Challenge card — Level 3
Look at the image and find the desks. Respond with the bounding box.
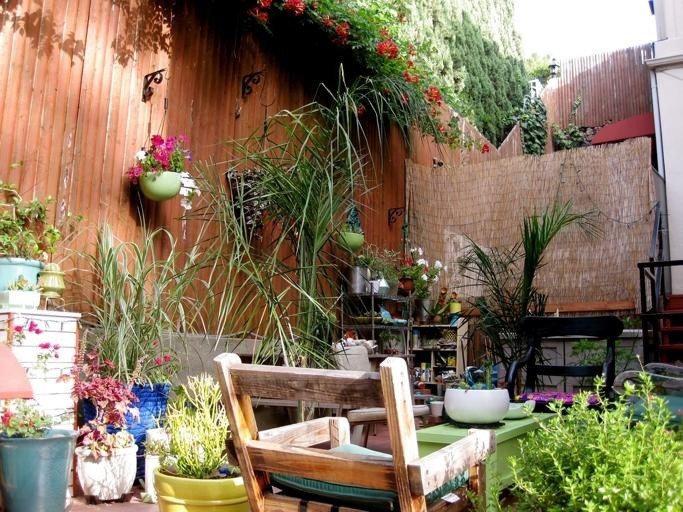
[415,411,561,511]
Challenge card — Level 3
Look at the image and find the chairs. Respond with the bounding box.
[505,314,626,424]
[331,343,432,448]
[212,351,498,512]
[612,361,682,437]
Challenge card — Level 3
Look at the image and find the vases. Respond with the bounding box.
[81,379,171,487]
[0,428,73,512]
[398,276,415,298]
[445,387,510,425]
[75,444,139,502]
[139,170,183,202]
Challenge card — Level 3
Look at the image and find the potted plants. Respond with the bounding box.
[137,370,252,512]
[336,219,366,253]
[2,273,43,310]
[0,157,84,290]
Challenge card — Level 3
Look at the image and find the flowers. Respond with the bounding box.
[47,197,218,391]
[0,409,51,439]
[400,246,449,299]
[124,132,194,186]
[59,341,142,460]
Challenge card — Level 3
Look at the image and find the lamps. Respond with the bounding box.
[0,342,33,415]
